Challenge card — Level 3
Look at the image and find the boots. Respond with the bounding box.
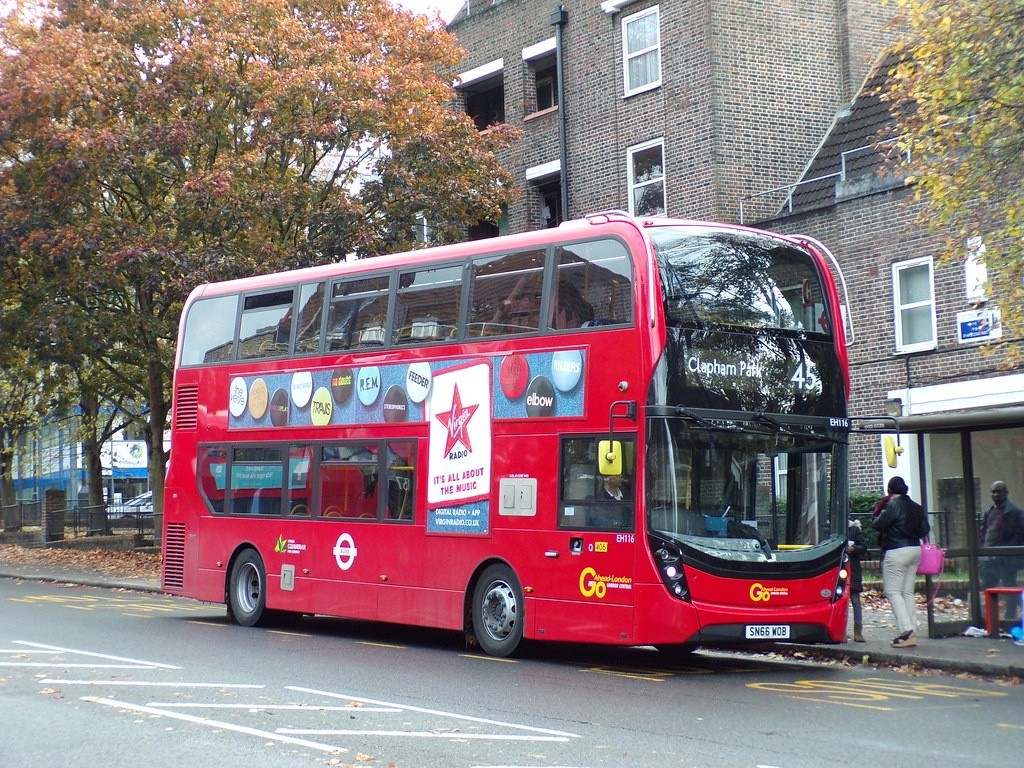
[854,624,866,642]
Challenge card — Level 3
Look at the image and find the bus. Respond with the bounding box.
[159,210,904,659]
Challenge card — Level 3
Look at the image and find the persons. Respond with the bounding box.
[872,476,931,648]
[980,480,1024,619]
[846,519,868,642]
[590,474,634,527]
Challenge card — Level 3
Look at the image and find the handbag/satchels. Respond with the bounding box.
[917,536,943,573]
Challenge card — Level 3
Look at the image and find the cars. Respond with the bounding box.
[108,491,154,521]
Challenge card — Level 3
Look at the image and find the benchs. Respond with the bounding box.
[984,585,1024,637]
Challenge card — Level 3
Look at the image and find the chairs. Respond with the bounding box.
[285,505,377,520]
[222,323,506,360]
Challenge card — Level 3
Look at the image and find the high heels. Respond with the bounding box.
[891,630,917,648]
